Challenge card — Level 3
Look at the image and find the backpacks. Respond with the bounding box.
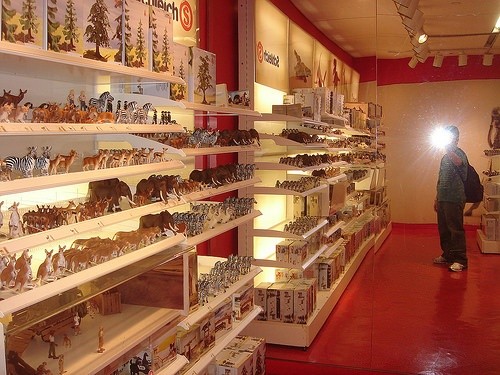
[452,148,483,202]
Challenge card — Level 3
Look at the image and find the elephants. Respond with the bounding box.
[288,132,312,144]
[189,162,237,186]
[139,210,179,233]
[87,174,182,213]
[215,128,263,147]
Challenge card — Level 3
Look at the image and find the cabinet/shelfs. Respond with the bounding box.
[375,104,394,256]
[475,147,500,254]
[247,110,331,354]
[346,120,375,259]
[101,85,267,375]
[0,37,194,375]
[287,115,351,302]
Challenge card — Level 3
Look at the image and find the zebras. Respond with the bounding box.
[3,146,52,178]
[89,92,156,123]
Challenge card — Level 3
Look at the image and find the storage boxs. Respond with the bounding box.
[117,202,268,375]
[256,232,351,325]
[481,214,497,239]
[276,86,367,123]
[0,0,218,115]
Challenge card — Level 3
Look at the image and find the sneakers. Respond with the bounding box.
[434,255,450,263]
[449,262,465,271]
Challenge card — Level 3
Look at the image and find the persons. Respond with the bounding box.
[433,125,468,272]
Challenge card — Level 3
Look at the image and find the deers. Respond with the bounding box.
[0,88,27,109]
[50,148,169,175]
[22,200,86,235]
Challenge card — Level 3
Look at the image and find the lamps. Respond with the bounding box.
[390,0,495,69]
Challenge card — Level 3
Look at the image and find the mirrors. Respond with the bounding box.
[206,0,500,375]
